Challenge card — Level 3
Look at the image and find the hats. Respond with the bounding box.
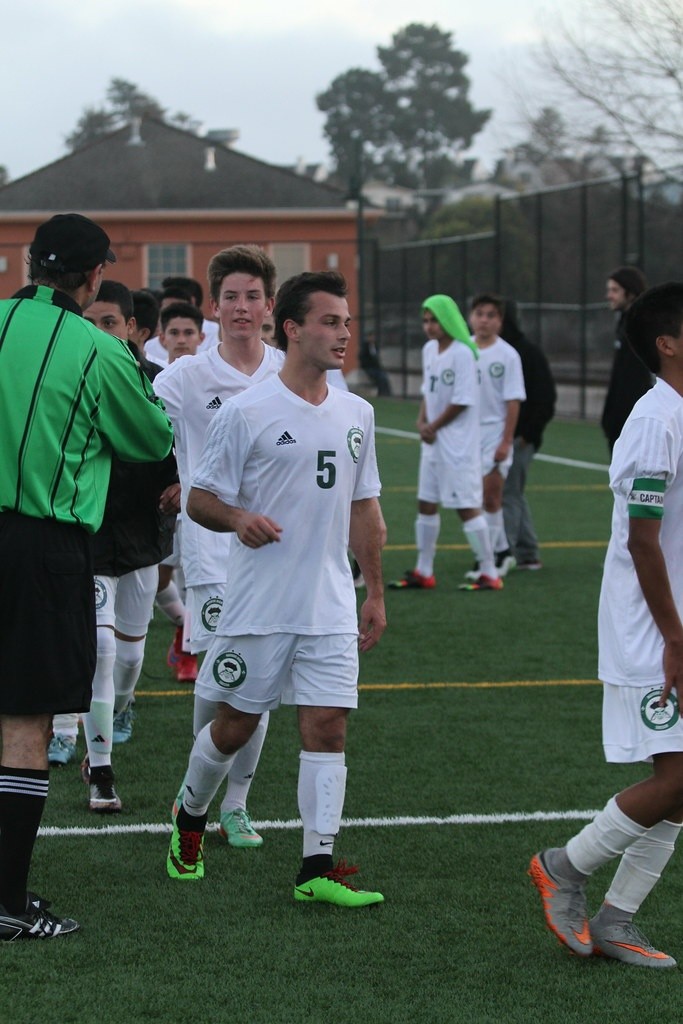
[28,213,118,268]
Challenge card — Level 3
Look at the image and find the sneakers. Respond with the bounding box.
[177,651,199,683]
[113,699,133,745]
[81,752,92,783]
[525,847,593,956]
[218,807,264,848]
[293,848,385,907]
[464,549,516,579]
[0,903,79,941]
[389,569,435,590]
[167,814,204,881]
[48,733,78,764]
[171,782,185,821]
[87,765,122,812]
[588,902,677,969]
[167,626,182,667]
[457,573,504,592]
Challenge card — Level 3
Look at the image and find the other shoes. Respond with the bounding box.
[515,558,542,571]
[354,568,368,589]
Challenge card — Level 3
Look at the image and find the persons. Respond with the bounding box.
[0,212,287,945]
[600,270,659,464]
[504,299,559,573]
[526,281,683,970]
[164,269,388,909]
[392,292,504,590]
[468,295,517,579]
[359,331,393,397]
